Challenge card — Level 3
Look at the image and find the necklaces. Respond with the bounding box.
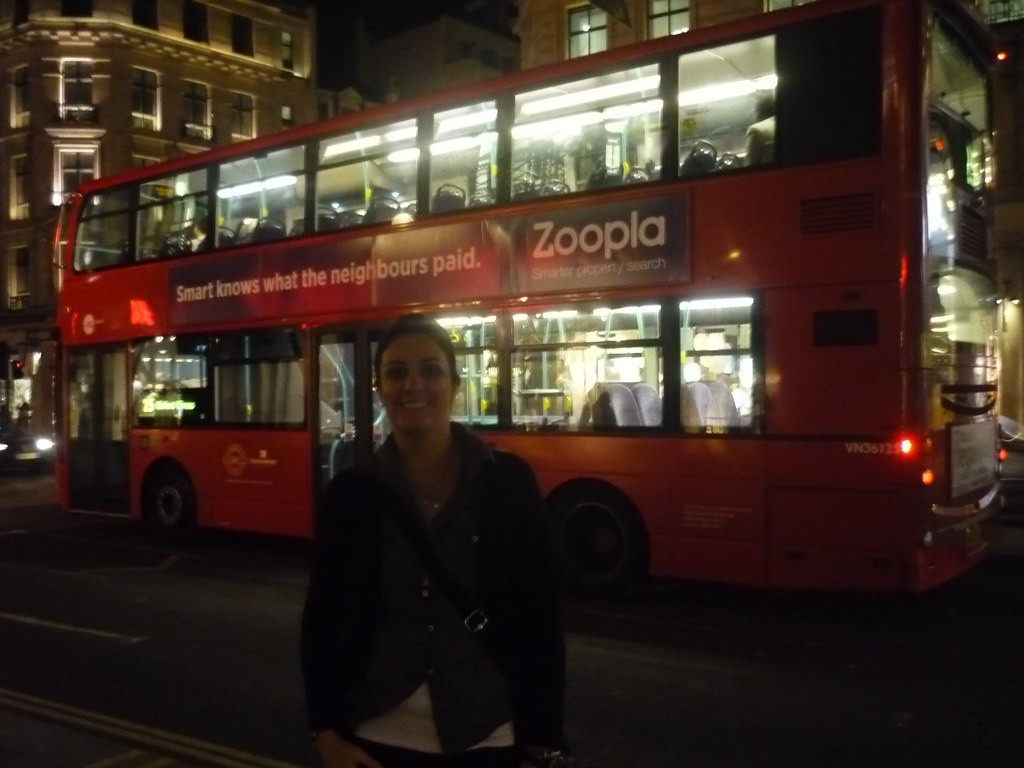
[419,497,442,510]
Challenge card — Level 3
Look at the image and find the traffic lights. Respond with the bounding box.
[10,361,24,380]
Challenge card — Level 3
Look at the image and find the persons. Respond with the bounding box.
[744,90,776,167]
[298,312,573,768]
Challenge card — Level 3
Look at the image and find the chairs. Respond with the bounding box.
[708,382,740,426]
[543,178,571,196]
[600,383,643,427]
[631,383,662,427]
[682,381,719,425]
[717,152,741,170]
[679,139,718,176]
[194,226,242,251]
[248,217,287,242]
[624,166,652,184]
[365,196,401,224]
[289,207,339,236]
[581,155,625,190]
[432,183,466,213]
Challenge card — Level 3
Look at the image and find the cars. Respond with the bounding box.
[995,415,1024,522]
[0,421,53,478]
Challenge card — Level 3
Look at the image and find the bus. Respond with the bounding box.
[54,0,1002,628]
